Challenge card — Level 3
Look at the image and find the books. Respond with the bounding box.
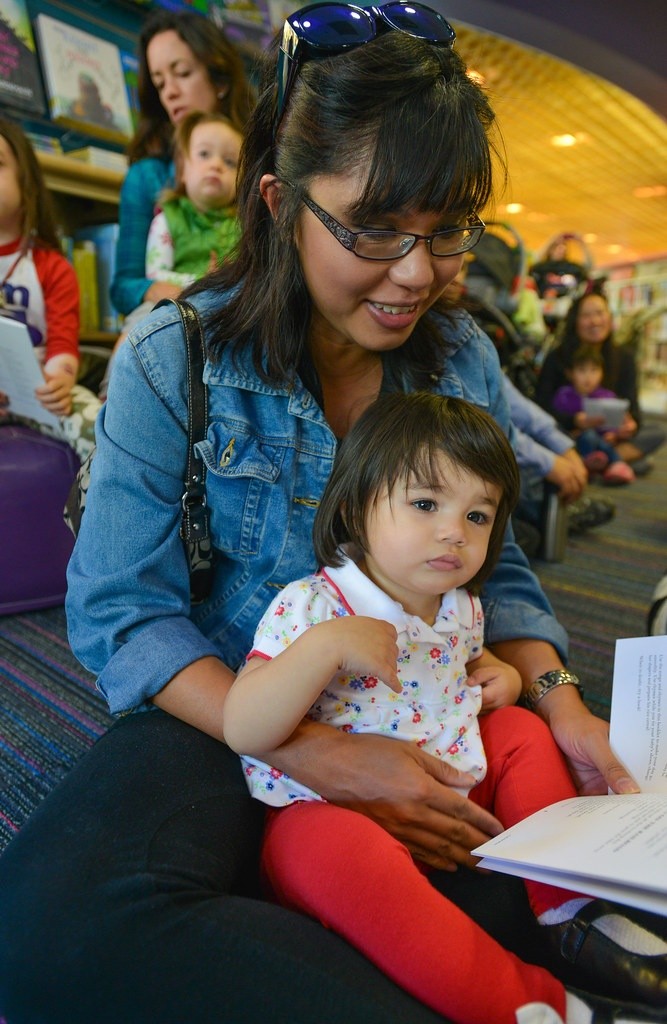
[472,633,667,917]
[0,0,47,117]
[26,132,129,170]
[36,12,135,144]
[57,223,125,333]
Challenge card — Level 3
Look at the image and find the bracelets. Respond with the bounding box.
[525,670,584,711]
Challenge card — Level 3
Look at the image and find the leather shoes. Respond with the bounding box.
[537,899,667,1016]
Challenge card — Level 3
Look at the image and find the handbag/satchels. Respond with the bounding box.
[65,297,209,593]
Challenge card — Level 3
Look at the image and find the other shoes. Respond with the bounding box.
[584,452,608,474]
[565,490,611,534]
[607,462,635,483]
[515,518,540,556]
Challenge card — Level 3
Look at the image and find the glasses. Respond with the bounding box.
[265,0,458,152]
[269,167,488,262]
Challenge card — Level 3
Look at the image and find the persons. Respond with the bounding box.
[504,373,614,562]
[109,9,258,313]
[0,119,103,533]
[533,291,667,475]
[551,350,638,483]
[97,111,247,403]
[222,393,667,1024]
[0,0,642,1024]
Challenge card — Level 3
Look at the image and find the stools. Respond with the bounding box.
[0,424,80,614]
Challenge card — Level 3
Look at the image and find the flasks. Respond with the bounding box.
[545,490,568,564]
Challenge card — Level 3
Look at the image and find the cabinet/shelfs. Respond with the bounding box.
[32,148,126,344]
